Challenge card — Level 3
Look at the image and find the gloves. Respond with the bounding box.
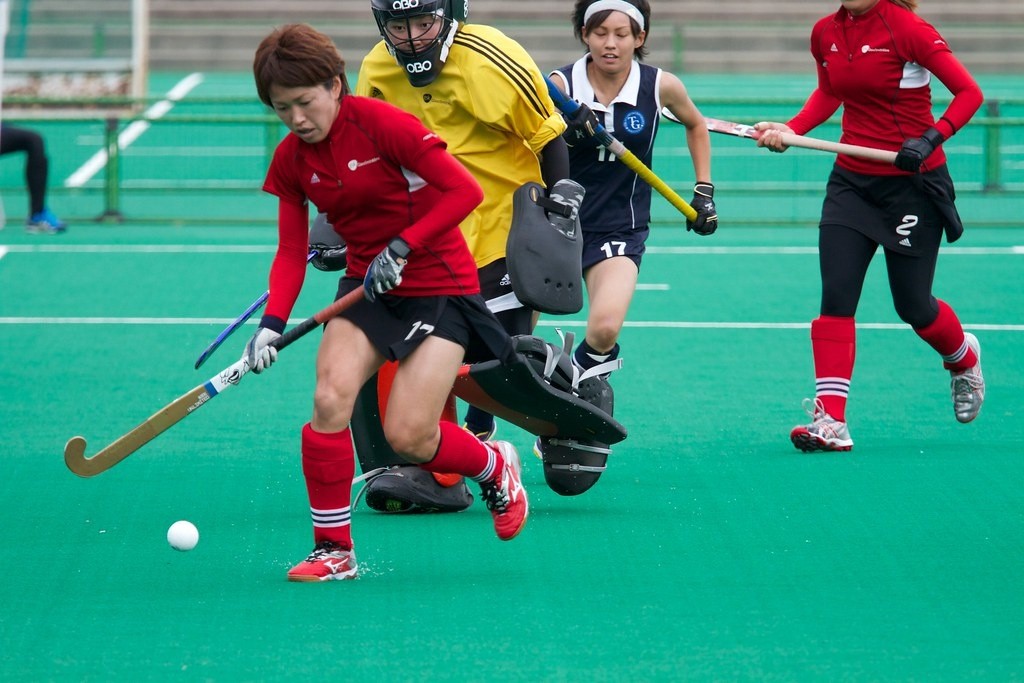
[363,238,411,302]
[894,128,941,174]
[685,182,716,234]
[232,316,288,385]
[561,98,598,147]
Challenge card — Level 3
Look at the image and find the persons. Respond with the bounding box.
[0,127,67,235]
[755,0,984,453]
[351,0,717,514]
[242,22,529,583]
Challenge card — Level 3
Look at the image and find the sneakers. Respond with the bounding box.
[29,207,66,234]
[288,540,358,581]
[951,332,984,422]
[532,344,620,461]
[479,440,528,541]
[790,397,853,451]
[460,416,498,443]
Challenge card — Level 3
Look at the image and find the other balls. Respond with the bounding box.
[166,519,200,552]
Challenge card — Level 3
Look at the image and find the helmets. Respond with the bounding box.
[370,0,467,89]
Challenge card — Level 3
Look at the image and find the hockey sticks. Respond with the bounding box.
[194,248,324,370]
[541,72,700,223]
[63,284,367,479]
[660,105,899,163]
